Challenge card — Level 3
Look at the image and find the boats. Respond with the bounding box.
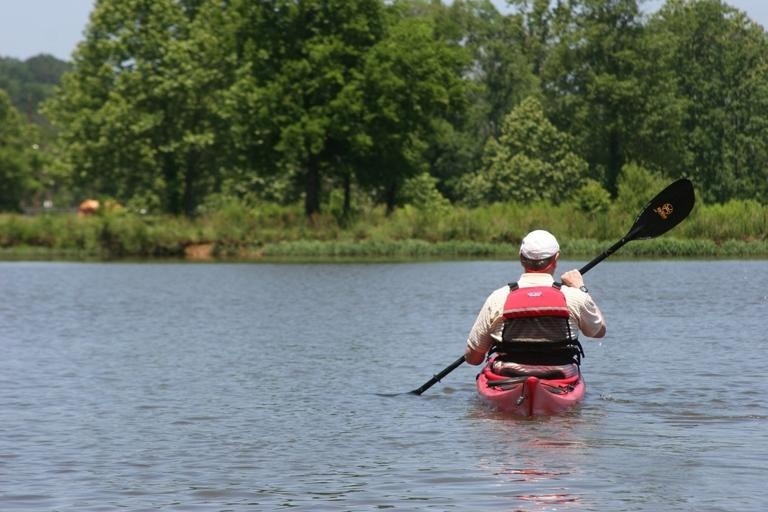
[476,354,585,421]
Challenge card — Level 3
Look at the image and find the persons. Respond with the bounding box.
[465,229,608,380]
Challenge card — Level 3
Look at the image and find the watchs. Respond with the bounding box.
[579,286,589,293]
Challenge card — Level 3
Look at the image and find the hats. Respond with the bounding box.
[518,230,562,261]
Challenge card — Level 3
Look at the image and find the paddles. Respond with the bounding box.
[408,179,695,396]
